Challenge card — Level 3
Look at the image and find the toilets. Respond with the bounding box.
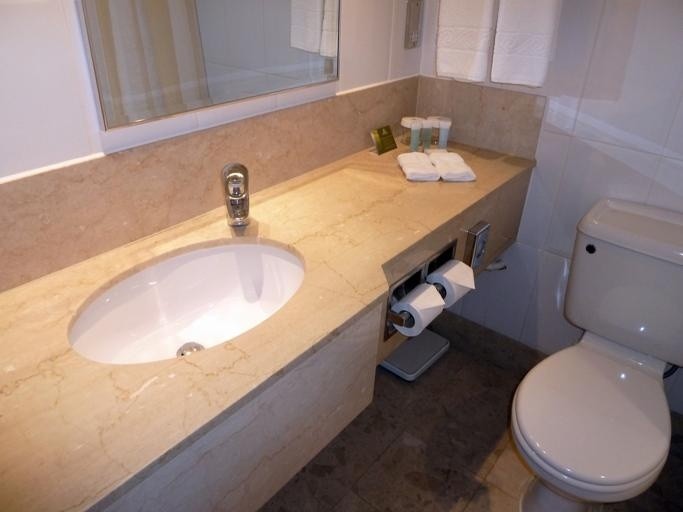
[509,195,683,510]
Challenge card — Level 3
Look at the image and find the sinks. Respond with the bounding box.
[65,235,305,363]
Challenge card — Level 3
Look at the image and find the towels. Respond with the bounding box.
[395,145,477,185]
[290,0,341,59]
[434,0,564,89]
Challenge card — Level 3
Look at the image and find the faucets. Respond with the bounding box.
[220,160,252,231]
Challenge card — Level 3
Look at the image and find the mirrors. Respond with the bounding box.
[71,0,345,133]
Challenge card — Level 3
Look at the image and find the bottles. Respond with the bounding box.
[400,115,452,151]
